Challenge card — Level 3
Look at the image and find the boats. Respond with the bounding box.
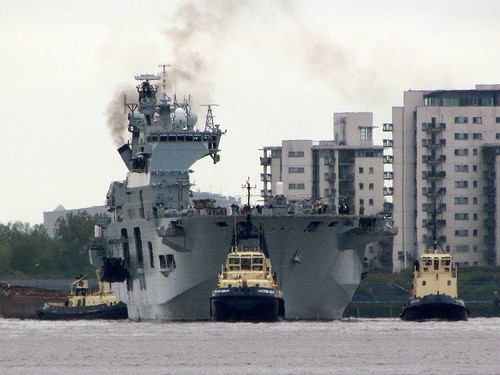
[400,252,470,321]
[209,243,284,323]
[35,266,128,321]
